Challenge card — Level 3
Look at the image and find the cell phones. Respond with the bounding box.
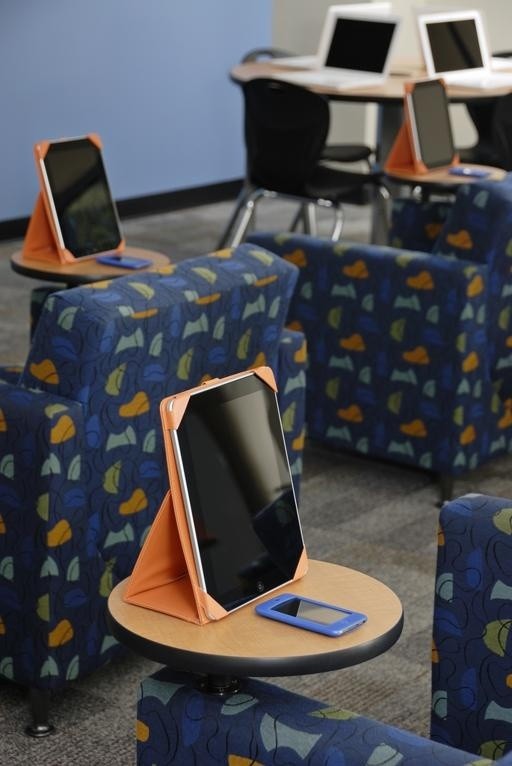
[450,167,490,177]
[255,593,368,637]
[97,255,153,270]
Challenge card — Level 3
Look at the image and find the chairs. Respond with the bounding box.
[0,242,310,734]
[242,50,378,241]
[136,490,512,766]
[215,76,376,249]
[239,174,512,509]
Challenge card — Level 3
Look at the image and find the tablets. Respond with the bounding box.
[406,78,458,174]
[168,366,309,619]
[36,134,126,262]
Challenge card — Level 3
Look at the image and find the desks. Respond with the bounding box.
[227,54,512,172]
[381,161,507,199]
[106,525,406,679]
[7,245,172,288]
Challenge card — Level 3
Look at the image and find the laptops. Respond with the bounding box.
[272,1,512,90]
